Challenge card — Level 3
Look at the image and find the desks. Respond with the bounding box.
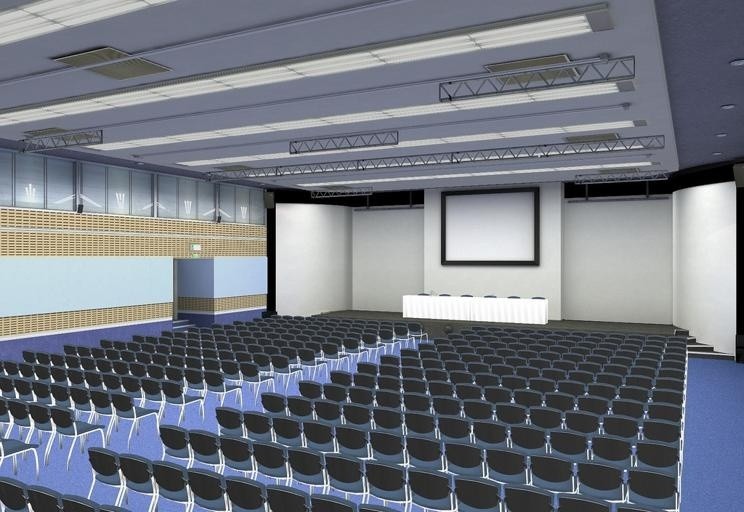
[401,295,548,325]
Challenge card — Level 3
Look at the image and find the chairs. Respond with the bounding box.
[1,316,687,510]
